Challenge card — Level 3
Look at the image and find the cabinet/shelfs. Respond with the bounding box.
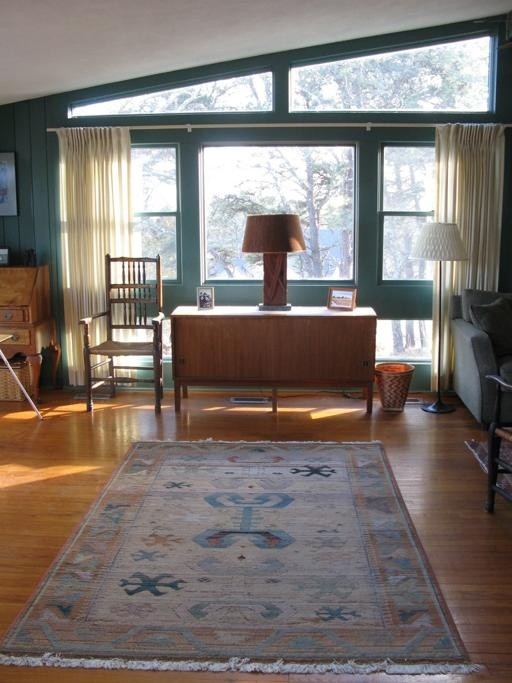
[169,305,377,413]
[0,264,61,403]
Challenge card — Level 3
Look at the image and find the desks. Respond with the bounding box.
[0,335,44,421]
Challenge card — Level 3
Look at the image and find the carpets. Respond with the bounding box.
[464,440,512,496]
[0,437,479,676]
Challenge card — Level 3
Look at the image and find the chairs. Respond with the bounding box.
[484,374,512,513]
[79,254,165,412]
[448,293,512,431]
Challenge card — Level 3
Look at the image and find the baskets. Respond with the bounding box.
[1,359,32,401]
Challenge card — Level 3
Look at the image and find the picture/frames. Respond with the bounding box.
[328,288,357,311]
[0,246,11,267]
[0,152,18,216]
[197,287,214,310]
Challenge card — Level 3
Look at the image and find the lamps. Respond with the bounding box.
[242,214,306,311]
[408,222,469,413]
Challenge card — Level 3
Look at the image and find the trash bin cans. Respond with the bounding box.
[374,362,415,411]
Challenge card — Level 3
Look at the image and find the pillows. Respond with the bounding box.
[463,289,499,322]
[469,297,512,356]
